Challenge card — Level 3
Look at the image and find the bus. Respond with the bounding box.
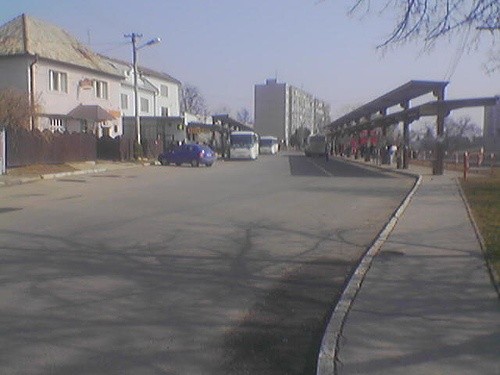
[259,136,279,155]
[229,131,259,160]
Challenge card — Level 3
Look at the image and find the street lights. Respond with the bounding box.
[133,37,161,146]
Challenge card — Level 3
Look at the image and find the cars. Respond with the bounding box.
[158,143,215,167]
